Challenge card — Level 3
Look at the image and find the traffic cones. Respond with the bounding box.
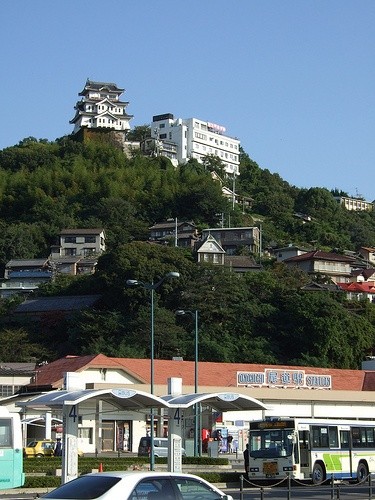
[99,463,104,472]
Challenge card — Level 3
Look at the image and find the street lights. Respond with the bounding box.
[174,309,198,459]
[125,271,180,471]
[167,217,177,247]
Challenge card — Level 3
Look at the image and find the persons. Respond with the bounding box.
[53,438,62,457]
[243,444,249,482]
[203,427,210,453]
[217,431,223,442]
[227,434,233,454]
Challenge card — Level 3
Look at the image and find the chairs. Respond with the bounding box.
[147,491,160,500]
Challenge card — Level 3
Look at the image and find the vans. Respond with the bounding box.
[138,437,186,457]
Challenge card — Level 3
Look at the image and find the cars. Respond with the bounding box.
[33,471,234,500]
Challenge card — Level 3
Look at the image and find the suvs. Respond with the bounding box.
[23,440,58,458]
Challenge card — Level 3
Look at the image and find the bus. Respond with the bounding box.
[0,406,25,491]
[247,416,375,487]
